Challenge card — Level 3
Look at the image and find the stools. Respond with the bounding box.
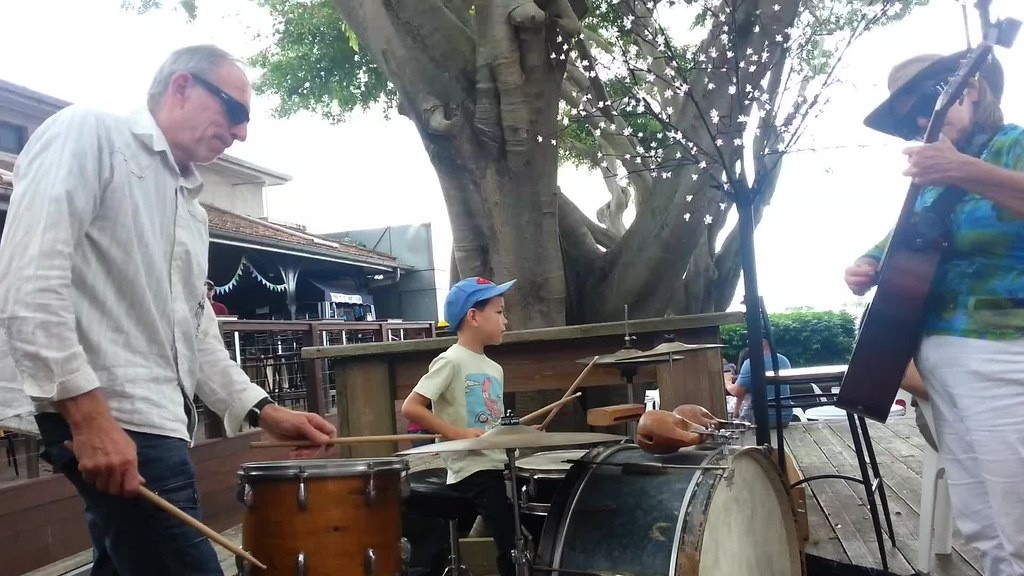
[403,466,481,576]
[243,330,373,412]
[746,295,895,576]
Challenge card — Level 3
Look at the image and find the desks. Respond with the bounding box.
[805,404,905,422]
[300,311,745,458]
[0,415,43,480]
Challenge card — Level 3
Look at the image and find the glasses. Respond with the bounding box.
[893,80,950,140]
[206,285,214,291]
[187,72,251,126]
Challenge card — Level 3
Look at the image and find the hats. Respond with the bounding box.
[863,53,1003,140]
[443,277,518,331]
[207,279,214,285]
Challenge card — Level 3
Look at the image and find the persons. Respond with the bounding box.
[724,330,794,430]
[844,47,1024,575]
[402,276,546,576]
[0,46,338,576]
[206,280,228,315]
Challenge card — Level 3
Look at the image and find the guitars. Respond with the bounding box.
[833,20,1001,424]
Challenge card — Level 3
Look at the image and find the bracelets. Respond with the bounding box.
[248,397,275,429]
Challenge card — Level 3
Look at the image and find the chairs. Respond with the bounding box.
[913,394,951,574]
[646,384,828,423]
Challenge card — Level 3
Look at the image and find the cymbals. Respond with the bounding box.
[614,342,730,362]
[575,349,686,364]
[397,420,630,453]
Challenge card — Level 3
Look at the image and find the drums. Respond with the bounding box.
[235,455,411,576]
[516,450,588,550]
[532,442,806,575]
